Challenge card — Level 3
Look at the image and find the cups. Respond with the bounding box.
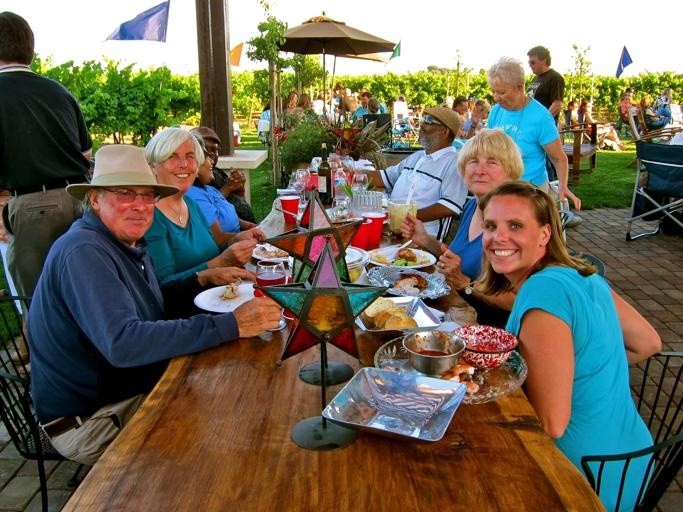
[269,142,417,250]
[282,275,298,321]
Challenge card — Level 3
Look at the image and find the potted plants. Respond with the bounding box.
[276,112,334,172]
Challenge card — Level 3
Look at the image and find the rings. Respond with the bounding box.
[440,263,445,270]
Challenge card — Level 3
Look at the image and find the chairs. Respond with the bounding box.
[582,350,683,511]
[626,108,683,168]
[637,105,681,143]
[625,141,683,242]
[0,293,86,512]
[559,122,605,186]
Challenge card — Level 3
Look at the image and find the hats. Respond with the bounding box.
[424,106,461,134]
[190,127,220,142]
[66,145,181,200]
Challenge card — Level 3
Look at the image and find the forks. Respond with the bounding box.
[386,239,412,262]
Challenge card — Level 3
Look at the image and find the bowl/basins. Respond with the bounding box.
[451,324,519,371]
[402,329,466,375]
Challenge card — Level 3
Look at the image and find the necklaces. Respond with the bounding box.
[164,197,184,225]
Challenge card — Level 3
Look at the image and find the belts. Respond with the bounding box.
[45,410,92,437]
[12,175,86,196]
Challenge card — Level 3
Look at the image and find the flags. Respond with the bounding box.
[615,45,633,79]
[103,1,170,42]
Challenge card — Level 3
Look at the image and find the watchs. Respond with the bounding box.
[464,278,476,296]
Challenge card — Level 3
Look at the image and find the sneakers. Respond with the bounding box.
[567,216,581,227]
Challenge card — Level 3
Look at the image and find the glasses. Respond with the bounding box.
[420,115,440,125]
[101,187,161,202]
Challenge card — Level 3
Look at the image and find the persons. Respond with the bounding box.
[24,144,283,464]
[486,56,582,210]
[337,106,468,243]
[182,130,258,246]
[0,11,92,324]
[400,129,524,329]
[142,128,269,287]
[190,126,257,228]
[259,80,683,153]
[528,47,565,181]
[477,182,662,512]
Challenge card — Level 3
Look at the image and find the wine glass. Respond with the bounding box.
[253,258,287,331]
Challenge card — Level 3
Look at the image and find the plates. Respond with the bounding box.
[366,247,438,270]
[192,283,254,315]
[352,296,441,338]
[322,366,467,442]
[343,245,369,273]
[367,267,452,300]
[372,330,529,406]
[250,242,290,263]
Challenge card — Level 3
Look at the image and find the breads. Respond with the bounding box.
[359,295,419,329]
[397,249,417,262]
